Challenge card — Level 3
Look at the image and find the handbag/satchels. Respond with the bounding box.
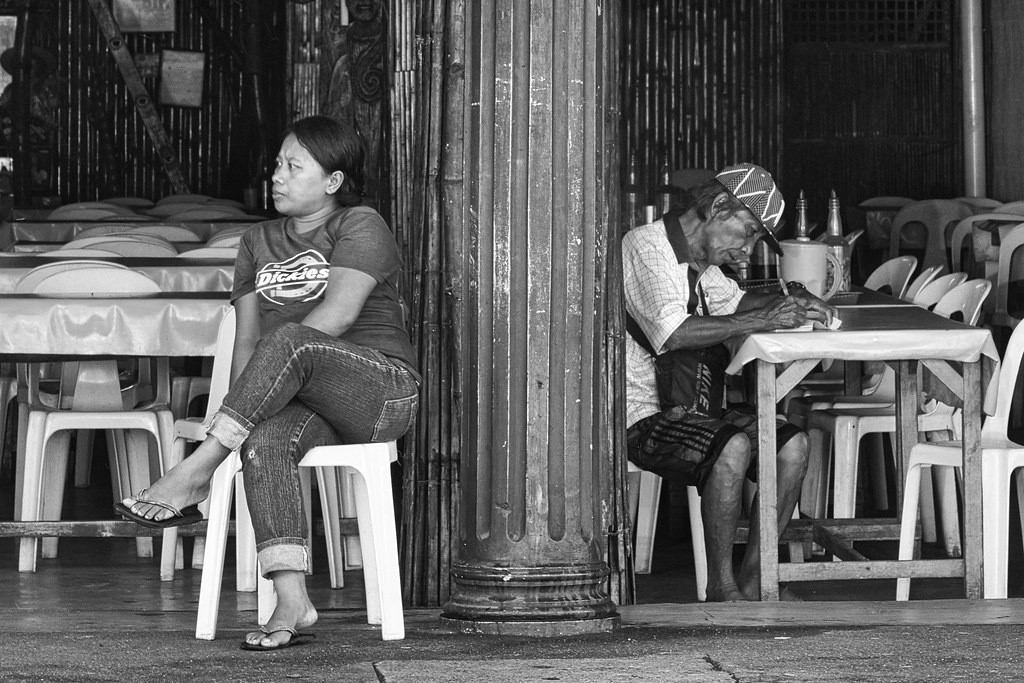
[655,350,725,418]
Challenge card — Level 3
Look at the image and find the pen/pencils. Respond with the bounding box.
[779,278,789,296]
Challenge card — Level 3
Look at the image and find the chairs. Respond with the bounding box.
[0,194,406,639]
[627,197,1024,607]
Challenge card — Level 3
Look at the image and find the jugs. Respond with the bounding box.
[779,235,843,302]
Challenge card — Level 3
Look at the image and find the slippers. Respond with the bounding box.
[113,490,203,528]
[240,624,315,651]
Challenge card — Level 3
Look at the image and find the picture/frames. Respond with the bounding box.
[111,0,176,34]
[155,47,205,109]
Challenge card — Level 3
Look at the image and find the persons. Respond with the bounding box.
[109,113,425,653]
[614,161,838,604]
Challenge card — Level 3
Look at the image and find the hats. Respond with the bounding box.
[715,163,784,257]
